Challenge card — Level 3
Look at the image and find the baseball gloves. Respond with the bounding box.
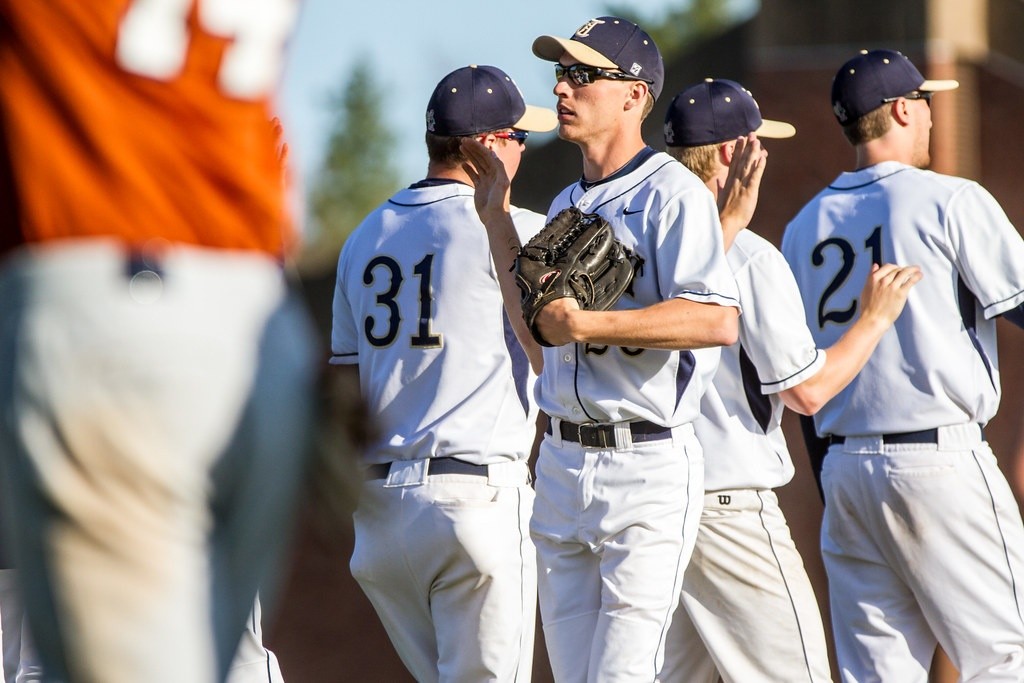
[506,205,642,348]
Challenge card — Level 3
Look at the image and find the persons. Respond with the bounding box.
[326,64,770,683]
[0,0,307,683]
[661,79,925,683]
[459,16,742,683]
[781,48,1024,683]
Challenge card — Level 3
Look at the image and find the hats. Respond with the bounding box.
[425,63,560,136]
[830,49,960,125]
[663,77,797,148]
[532,15,665,103]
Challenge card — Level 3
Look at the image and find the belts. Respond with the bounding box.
[823,428,938,446]
[545,416,672,449]
[365,456,488,482]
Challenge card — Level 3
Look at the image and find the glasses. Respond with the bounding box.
[555,63,655,86]
[881,91,935,108]
[477,126,529,146]
